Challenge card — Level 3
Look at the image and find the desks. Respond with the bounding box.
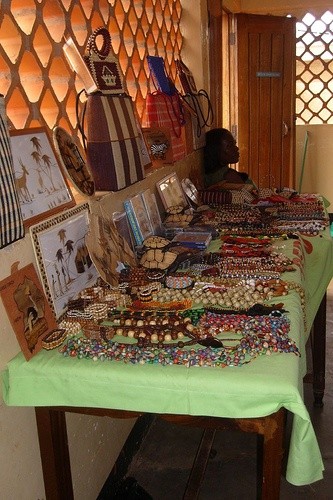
[0,195,333,499]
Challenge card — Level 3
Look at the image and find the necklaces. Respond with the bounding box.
[60,187,331,370]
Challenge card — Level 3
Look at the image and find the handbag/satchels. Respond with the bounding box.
[0,93,25,249]
[174,58,199,96]
[81,26,126,96]
[146,55,179,97]
[146,91,187,165]
[181,89,214,151]
[86,90,146,193]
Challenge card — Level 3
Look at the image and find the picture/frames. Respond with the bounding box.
[8,125,76,230]
[141,189,162,237]
[124,194,152,246]
[156,171,187,215]
[0,262,57,362]
[29,203,102,323]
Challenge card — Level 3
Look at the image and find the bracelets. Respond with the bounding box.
[41,201,220,352]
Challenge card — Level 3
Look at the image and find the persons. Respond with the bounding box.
[203,128,258,189]
